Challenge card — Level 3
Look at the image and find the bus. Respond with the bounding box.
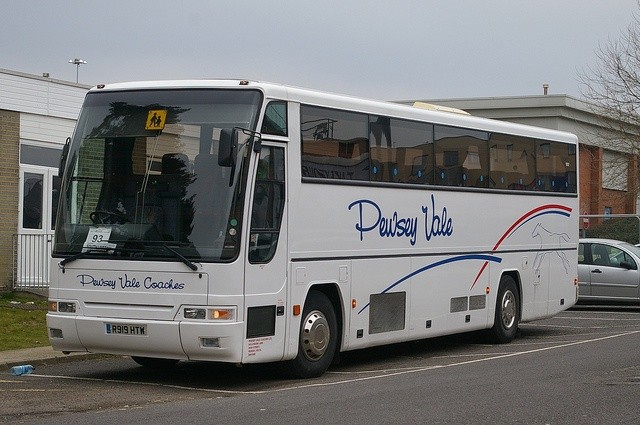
[45,78,579,379]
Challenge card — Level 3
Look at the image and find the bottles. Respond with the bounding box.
[10,365,38,376]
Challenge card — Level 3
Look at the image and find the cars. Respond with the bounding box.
[578,238,636,304]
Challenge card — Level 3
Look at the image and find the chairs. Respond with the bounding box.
[525,174,542,192]
[127,188,164,230]
[554,176,569,195]
[375,161,398,181]
[541,175,557,191]
[193,153,222,181]
[402,162,428,185]
[508,171,524,190]
[157,153,188,177]
[466,167,483,188]
[489,171,508,189]
[441,164,464,188]
